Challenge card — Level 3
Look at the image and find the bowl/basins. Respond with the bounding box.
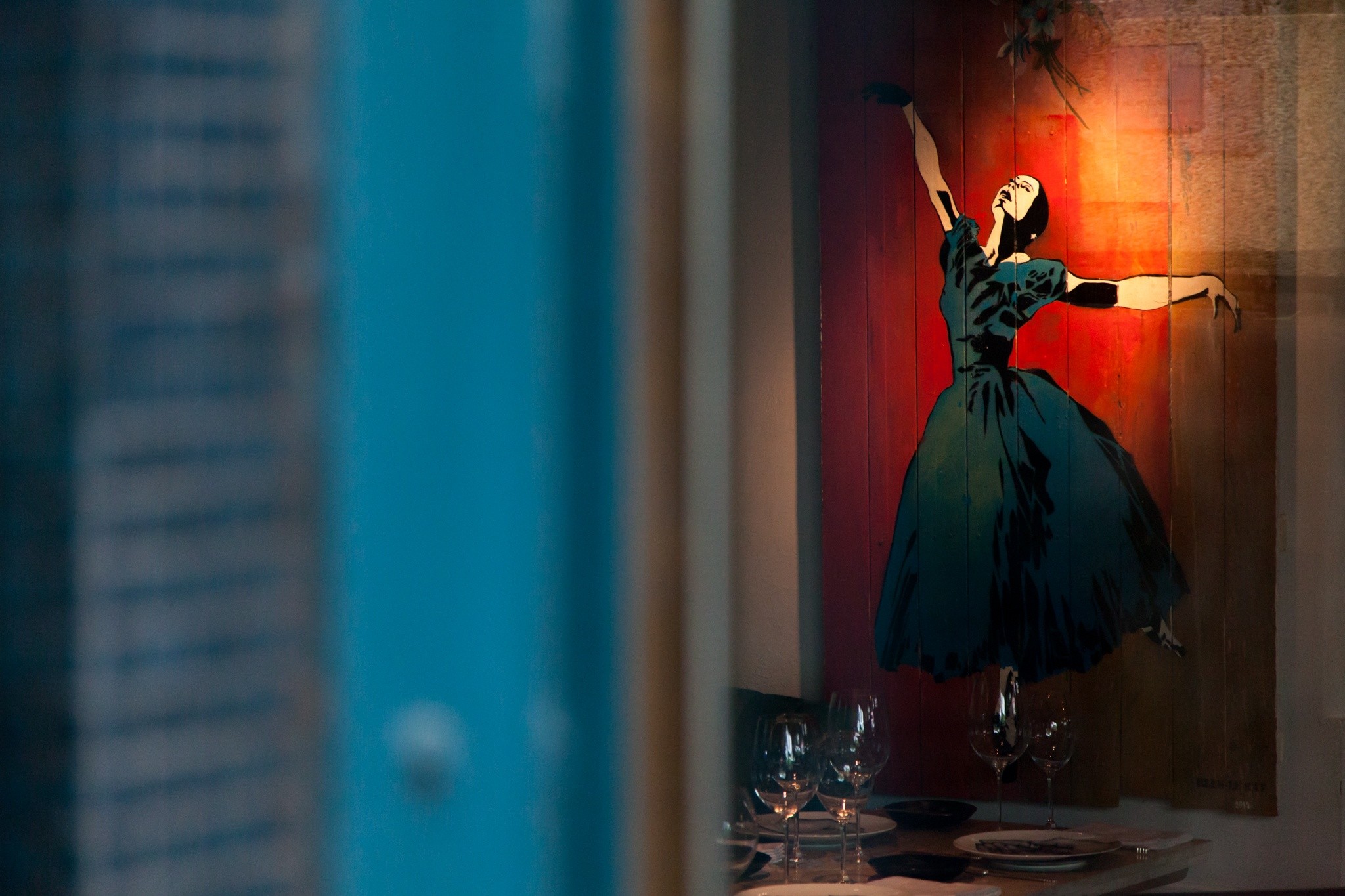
[867,854,969,883]
[881,799,977,830]
[726,845,771,884]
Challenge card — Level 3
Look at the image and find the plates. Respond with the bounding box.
[736,883,902,896]
[867,875,1002,896]
[731,811,897,847]
[954,830,1120,870]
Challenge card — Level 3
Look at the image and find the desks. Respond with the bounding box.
[733,811,1215,896]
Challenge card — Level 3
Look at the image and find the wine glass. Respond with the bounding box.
[1025,682,1075,829]
[769,721,816,873]
[749,715,826,884]
[817,764,875,884]
[966,674,1032,829]
[827,688,890,876]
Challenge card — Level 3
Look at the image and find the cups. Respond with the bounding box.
[725,786,758,896]
